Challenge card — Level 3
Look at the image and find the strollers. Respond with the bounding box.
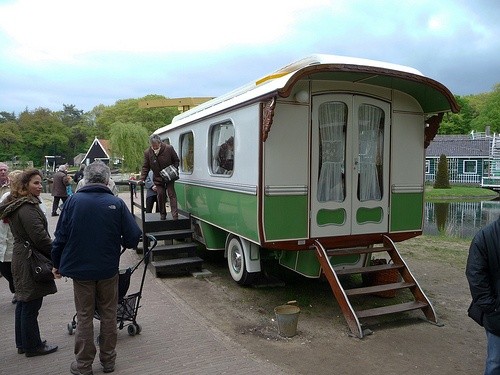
[65,235,158,346]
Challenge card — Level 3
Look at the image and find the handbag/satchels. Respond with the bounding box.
[159,165,179,183]
[29,248,55,281]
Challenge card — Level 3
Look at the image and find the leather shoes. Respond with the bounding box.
[19,339,46,353]
[25,344,58,357]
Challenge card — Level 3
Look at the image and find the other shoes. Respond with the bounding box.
[52,212,59,216]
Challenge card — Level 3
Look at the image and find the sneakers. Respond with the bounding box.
[173,216,178,220]
[70,361,93,375]
[161,214,166,220]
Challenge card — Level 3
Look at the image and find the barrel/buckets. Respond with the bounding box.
[274,300,301,338]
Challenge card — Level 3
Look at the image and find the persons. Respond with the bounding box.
[464,215,500,375]
[128,173,137,198]
[137,134,180,223]
[41,167,47,193]
[73,163,86,185]
[0,167,59,358]
[51,165,70,216]
[0,162,10,198]
[144,168,161,214]
[63,162,69,174]
[51,160,142,375]
[45,169,52,193]
[0,169,28,303]
